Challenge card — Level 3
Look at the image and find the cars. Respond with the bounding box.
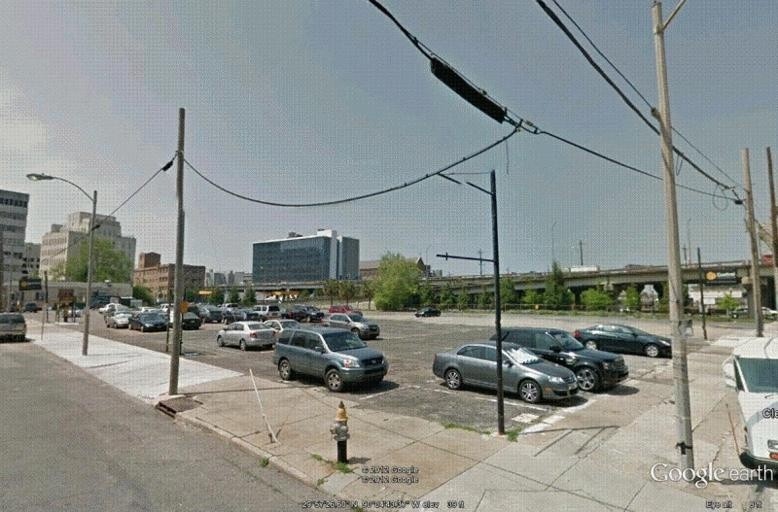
[22,303,38,313]
[97,300,390,394]
[572,323,673,361]
[414,306,442,319]
[60,306,81,318]
[430,339,579,406]
[728,304,777,321]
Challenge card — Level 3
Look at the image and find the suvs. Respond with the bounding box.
[0,312,27,343]
[489,327,630,393]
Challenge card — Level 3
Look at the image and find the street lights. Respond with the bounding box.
[686,218,692,266]
[27,170,99,358]
[549,221,558,255]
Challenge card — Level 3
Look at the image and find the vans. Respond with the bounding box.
[721,334,778,479]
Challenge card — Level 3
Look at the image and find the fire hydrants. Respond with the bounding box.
[329,401,350,464]
